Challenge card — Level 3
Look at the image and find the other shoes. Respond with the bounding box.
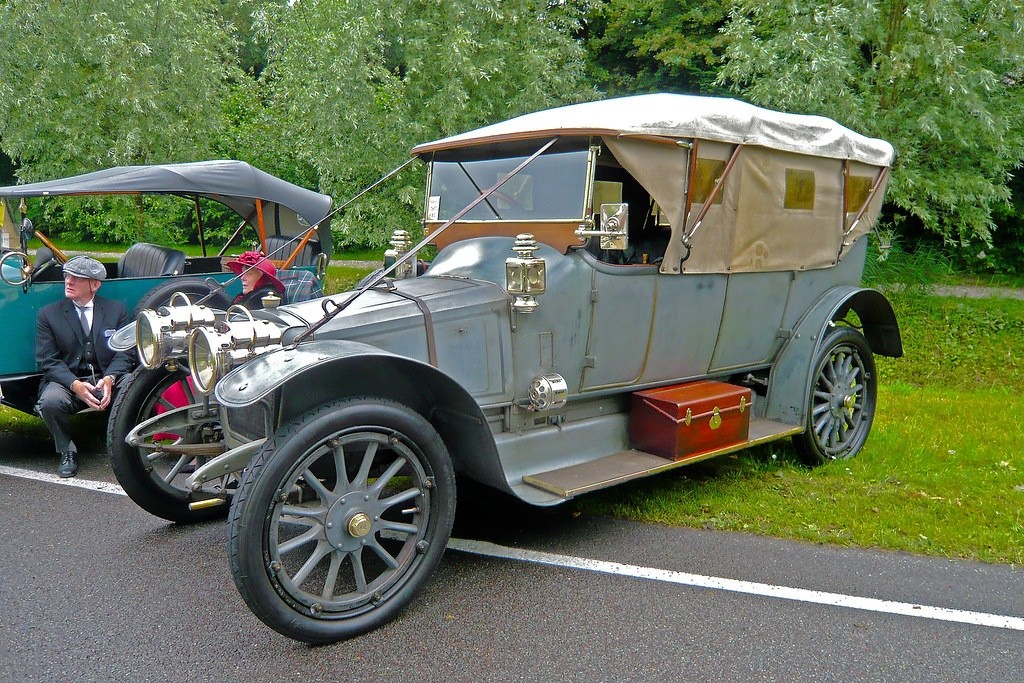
[178,462,195,473]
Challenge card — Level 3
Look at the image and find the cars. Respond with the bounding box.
[2,160,335,443]
[107,93,903,643]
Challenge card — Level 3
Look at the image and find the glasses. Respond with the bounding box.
[63,272,90,281]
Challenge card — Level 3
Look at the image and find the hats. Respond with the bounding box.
[63,255,107,282]
[226,251,286,295]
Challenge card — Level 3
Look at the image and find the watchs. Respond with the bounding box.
[112,377,115,386]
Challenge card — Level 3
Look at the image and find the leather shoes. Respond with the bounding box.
[57,450,79,478]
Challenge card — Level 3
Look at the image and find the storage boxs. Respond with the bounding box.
[629,380,754,462]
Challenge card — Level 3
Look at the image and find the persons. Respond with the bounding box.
[35,256,138,478]
[152,250,286,472]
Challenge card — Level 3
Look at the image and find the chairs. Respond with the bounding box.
[117,243,185,279]
[260,233,322,267]
